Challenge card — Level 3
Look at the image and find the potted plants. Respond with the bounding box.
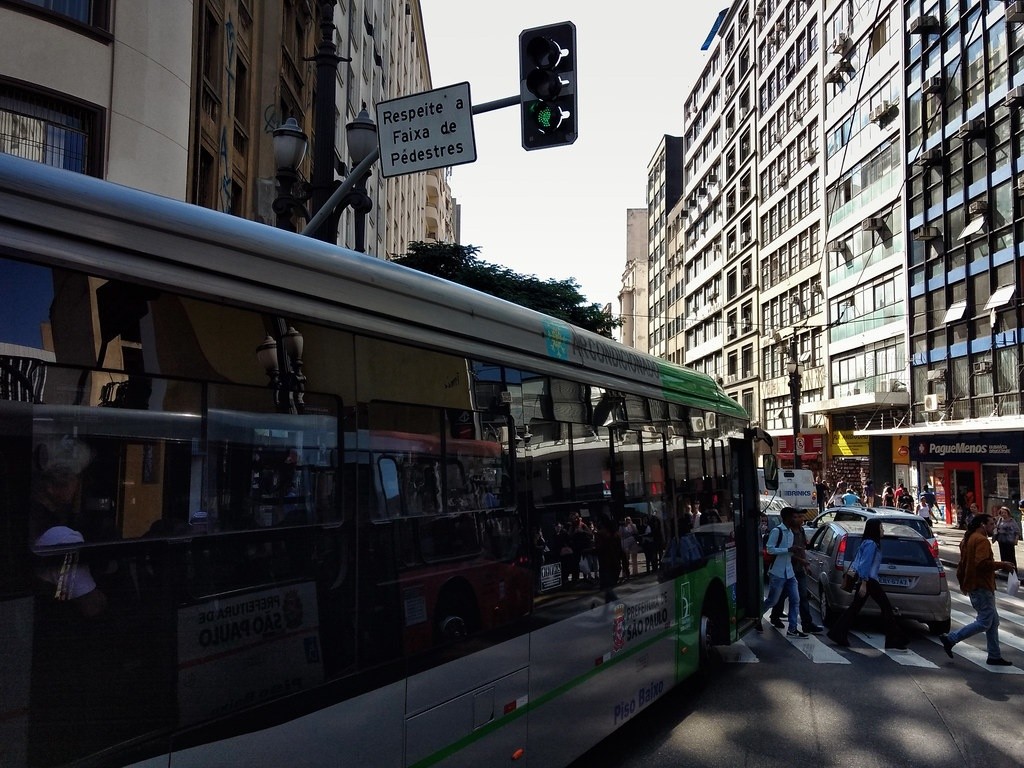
[910,16,934,34]
[1005,1,1024,23]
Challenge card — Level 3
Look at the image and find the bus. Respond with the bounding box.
[512,432,747,540]
[0,149,779,768]
[1,401,535,725]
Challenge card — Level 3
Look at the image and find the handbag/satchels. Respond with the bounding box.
[839,570,859,593]
[992,518,1002,543]
[1007,569,1020,596]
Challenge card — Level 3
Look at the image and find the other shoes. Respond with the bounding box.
[827,633,850,648]
[939,635,954,658]
[804,623,824,634]
[887,640,910,648]
[770,615,785,627]
[987,657,1013,666]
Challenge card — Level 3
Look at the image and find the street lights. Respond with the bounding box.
[271,0,378,253]
[784,327,804,468]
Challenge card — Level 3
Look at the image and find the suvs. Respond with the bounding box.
[801,521,952,634]
[800,505,939,558]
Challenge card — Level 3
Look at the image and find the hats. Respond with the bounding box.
[796,507,808,514]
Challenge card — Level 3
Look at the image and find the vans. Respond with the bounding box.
[757,469,818,524]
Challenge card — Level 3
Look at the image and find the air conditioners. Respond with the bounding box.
[832,33,846,53]
[1006,86,1024,105]
[875,100,889,119]
[835,62,849,72]
[742,318,749,329]
[813,284,822,293]
[928,370,944,379]
[869,112,878,124]
[847,389,860,396]
[774,133,780,143]
[756,7,764,15]
[880,379,899,393]
[973,362,992,374]
[826,240,845,251]
[712,243,720,251]
[825,74,840,83]
[792,111,801,121]
[864,219,883,231]
[969,201,987,214]
[707,268,748,301]
[769,330,774,339]
[959,120,984,139]
[920,150,941,166]
[789,297,799,304]
[804,147,812,160]
[777,174,787,185]
[913,227,937,241]
[925,394,945,413]
[674,258,678,266]
[664,268,670,275]
[728,371,753,383]
[921,77,940,93]
[751,421,760,428]
[1017,174,1024,196]
[741,232,749,244]
[730,242,735,254]
[693,307,698,312]
[678,254,682,263]
[727,326,734,336]
[713,374,719,382]
[692,22,751,208]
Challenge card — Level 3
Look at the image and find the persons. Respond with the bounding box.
[881,482,914,512]
[533,511,619,603]
[28,436,118,549]
[815,476,861,515]
[966,503,981,523]
[619,499,719,582]
[827,519,907,651]
[938,514,1016,666]
[919,485,942,516]
[916,496,938,527]
[755,506,824,638]
[994,506,1020,574]
[863,479,876,507]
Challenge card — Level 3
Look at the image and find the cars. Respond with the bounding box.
[734,494,792,532]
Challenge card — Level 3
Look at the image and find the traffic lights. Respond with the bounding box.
[518,20,577,152]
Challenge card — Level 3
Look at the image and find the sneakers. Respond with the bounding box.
[787,629,808,639]
